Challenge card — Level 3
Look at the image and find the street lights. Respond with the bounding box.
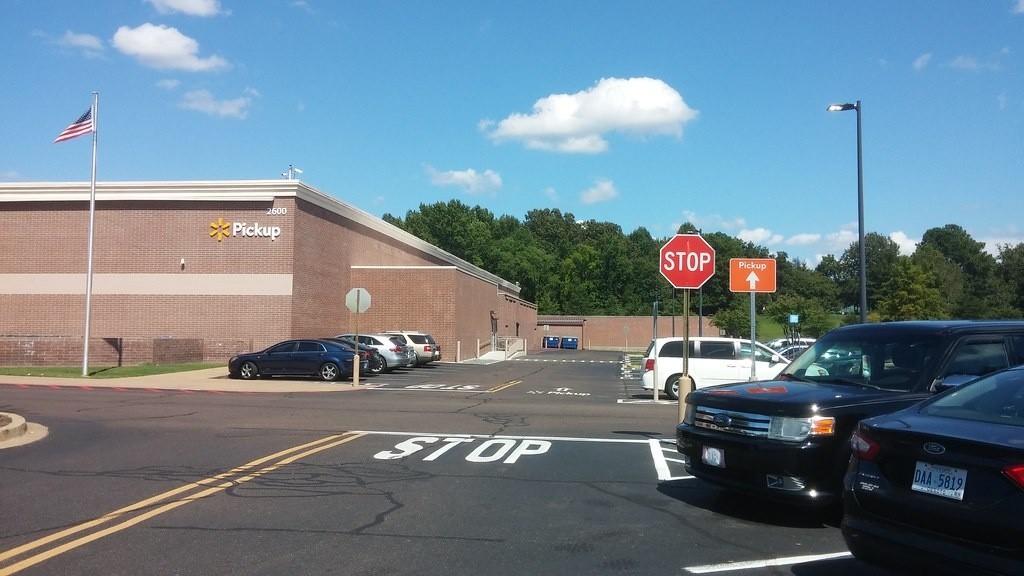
[826,99,870,324]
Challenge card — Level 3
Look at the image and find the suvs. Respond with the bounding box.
[642,337,829,399]
[675,320,1024,522]
[379,329,443,368]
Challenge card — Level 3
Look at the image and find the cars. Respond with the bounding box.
[322,337,382,379]
[840,363,1024,576]
[229,339,371,382]
[736,338,865,378]
[333,332,417,374]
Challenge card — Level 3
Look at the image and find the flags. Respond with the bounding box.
[52,105,94,144]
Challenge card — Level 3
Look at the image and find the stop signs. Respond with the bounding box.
[660,234,716,289]
